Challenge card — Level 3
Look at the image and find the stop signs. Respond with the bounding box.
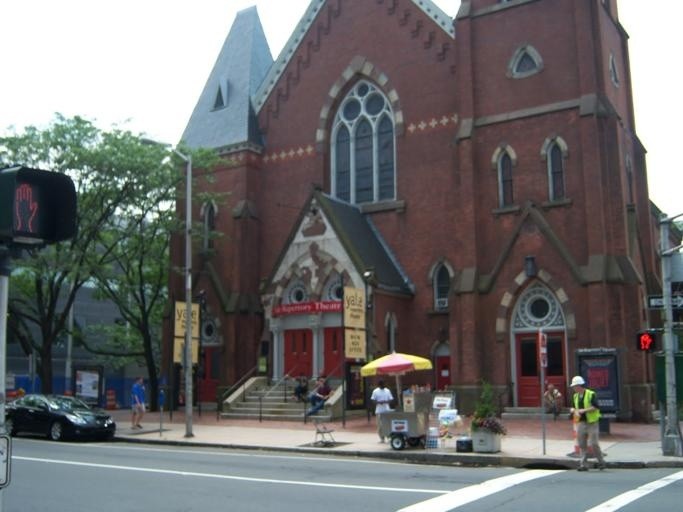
[536,333,548,366]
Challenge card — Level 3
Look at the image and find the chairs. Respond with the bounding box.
[309,415,336,446]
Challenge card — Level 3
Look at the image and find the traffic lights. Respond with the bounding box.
[636,331,655,352]
[1,165,79,251]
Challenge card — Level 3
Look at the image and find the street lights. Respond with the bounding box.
[137,136,195,439]
[362,266,377,424]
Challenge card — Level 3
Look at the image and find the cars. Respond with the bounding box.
[6,393,114,442]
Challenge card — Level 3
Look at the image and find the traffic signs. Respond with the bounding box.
[647,294,683,311]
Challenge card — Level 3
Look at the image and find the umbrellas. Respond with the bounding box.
[358,349,434,408]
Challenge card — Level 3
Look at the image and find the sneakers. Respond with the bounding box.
[577,466,590,471]
[599,461,606,470]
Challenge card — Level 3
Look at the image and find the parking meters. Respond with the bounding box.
[158,388,164,436]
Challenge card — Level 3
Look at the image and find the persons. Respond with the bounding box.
[290,377,302,402]
[297,376,309,402]
[129,376,146,431]
[300,378,334,417]
[544,383,563,416]
[368,378,395,443]
[302,367,328,403]
[569,375,607,472]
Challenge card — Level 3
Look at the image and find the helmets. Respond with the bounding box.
[570,376,586,388]
